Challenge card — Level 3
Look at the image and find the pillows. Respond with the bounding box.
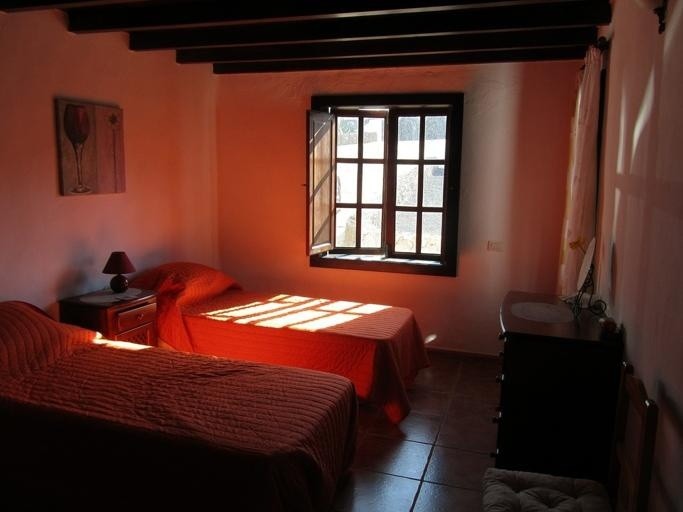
[131,261,249,303]
[1,295,111,388]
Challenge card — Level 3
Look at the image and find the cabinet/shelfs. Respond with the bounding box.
[488,289,627,512]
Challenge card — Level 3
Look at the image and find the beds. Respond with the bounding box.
[4,338,358,511]
[159,290,415,414]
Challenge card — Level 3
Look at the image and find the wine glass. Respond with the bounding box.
[64,104,91,196]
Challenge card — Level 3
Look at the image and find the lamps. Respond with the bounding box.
[101,251,136,293]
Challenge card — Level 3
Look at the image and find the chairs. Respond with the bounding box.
[481,362,660,512]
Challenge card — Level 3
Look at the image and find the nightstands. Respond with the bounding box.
[57,288,159,348]
